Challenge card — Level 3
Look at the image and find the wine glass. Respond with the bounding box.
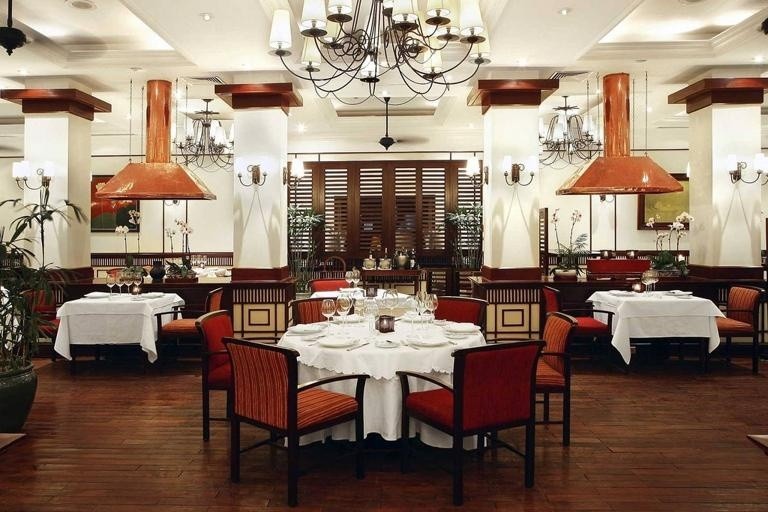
[186,255,207,269]
[337,290,438,333]
[346,269,361,289]
[321,299,336,335]
[107,272,142,299]
[642,269,660,299]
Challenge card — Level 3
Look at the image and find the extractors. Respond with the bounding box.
[95,78,216,201]
[550,70,683,197]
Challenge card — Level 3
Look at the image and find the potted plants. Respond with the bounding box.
[0,176,86,440]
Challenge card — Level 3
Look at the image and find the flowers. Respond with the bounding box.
[642,212,695,278]
[549,206,592,276]
[114,207,196,280]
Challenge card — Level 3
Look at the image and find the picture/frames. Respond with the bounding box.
[635,171,689,232]
[91,172,141,237]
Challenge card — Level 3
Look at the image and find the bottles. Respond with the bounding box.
[362,246,393,271]
[410,249,418,271]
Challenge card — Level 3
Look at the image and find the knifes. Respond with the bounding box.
[346,342,369,351]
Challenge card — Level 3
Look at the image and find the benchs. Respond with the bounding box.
[154,288,224,375]
[678,284,765,376]
[19,285,67,364]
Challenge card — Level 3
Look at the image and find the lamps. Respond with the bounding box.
[169,94,236,171]
[235,161,268,187]
[10,157,54,192]
[500,154,535,188]
[454,153,488,187]
[727,153,768,187]
[536,92,603,163]
[278,155,308,188]
[262,0,496,106]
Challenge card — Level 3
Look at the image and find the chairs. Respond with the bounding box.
[307,277,359,295]
[217,331,370,505]
[397,336,547,507]
[196,307,235,449]
[433,294,488,341]
[541,285,616,375]
[520,307,580,449]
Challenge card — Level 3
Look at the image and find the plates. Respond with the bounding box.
[319,337,357,351]
[287,324,319,334]
[665,290,693,297]
[437,319,481,338]
[374,339,398,348]
[141,292,164,299]
[608,289,634,298]
[83,292,108,299]
[405,330,448,347]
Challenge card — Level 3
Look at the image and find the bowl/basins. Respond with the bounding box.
[601,249,640,259]
[214,270,227,277]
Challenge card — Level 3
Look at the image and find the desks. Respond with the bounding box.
[361,266,423,294]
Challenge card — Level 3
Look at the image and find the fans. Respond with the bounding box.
[0,0,109,66]
[366,96,428,152]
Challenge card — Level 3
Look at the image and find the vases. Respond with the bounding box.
[549,267,581,281]
